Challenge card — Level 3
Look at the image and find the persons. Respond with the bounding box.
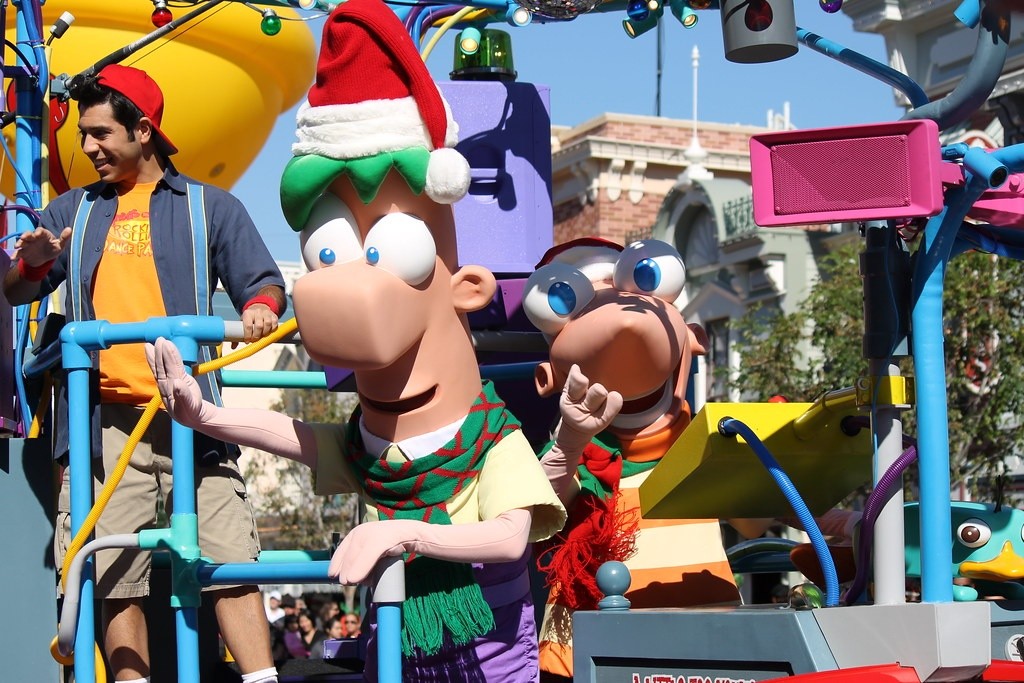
[4,64,288,683]
[267,589,362,660]
[523,237,869,608]
[143,0,566,683]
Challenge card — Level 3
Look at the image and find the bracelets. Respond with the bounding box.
[243,295,280,316]
[17,259,56,281]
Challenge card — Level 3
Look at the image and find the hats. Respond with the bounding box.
[95,65,179,157]
[269,589,281,601]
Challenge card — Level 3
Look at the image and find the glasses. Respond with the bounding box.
[344,620,358,624]
